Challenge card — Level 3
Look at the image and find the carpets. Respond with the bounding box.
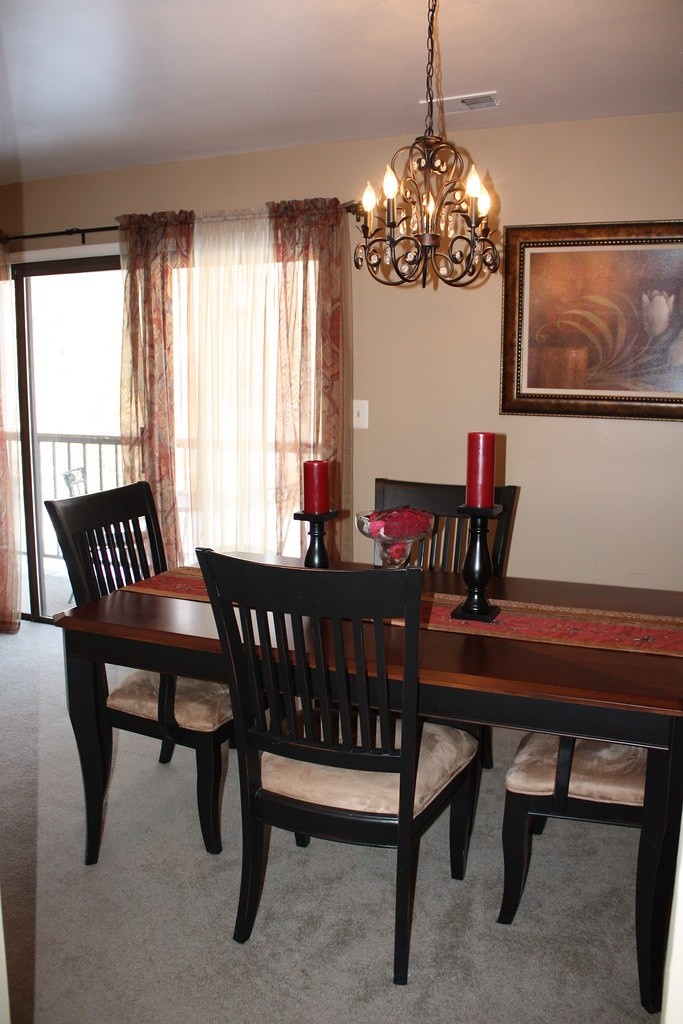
[35,620,680,1024]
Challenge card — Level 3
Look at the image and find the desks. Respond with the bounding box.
[53,544,682,1014]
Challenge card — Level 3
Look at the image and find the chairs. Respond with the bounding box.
[196,547,483,984]
[43,482,310,864]
[495,731,674,924]
[375,477,518,575]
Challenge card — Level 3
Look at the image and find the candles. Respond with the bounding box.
[303,460,330,515]
[466,431,496,508]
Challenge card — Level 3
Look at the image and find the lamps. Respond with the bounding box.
[338,0,498,290]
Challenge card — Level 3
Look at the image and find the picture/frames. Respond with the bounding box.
[499,218,682,423]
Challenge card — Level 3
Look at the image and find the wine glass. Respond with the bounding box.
[355,510,435,570]
